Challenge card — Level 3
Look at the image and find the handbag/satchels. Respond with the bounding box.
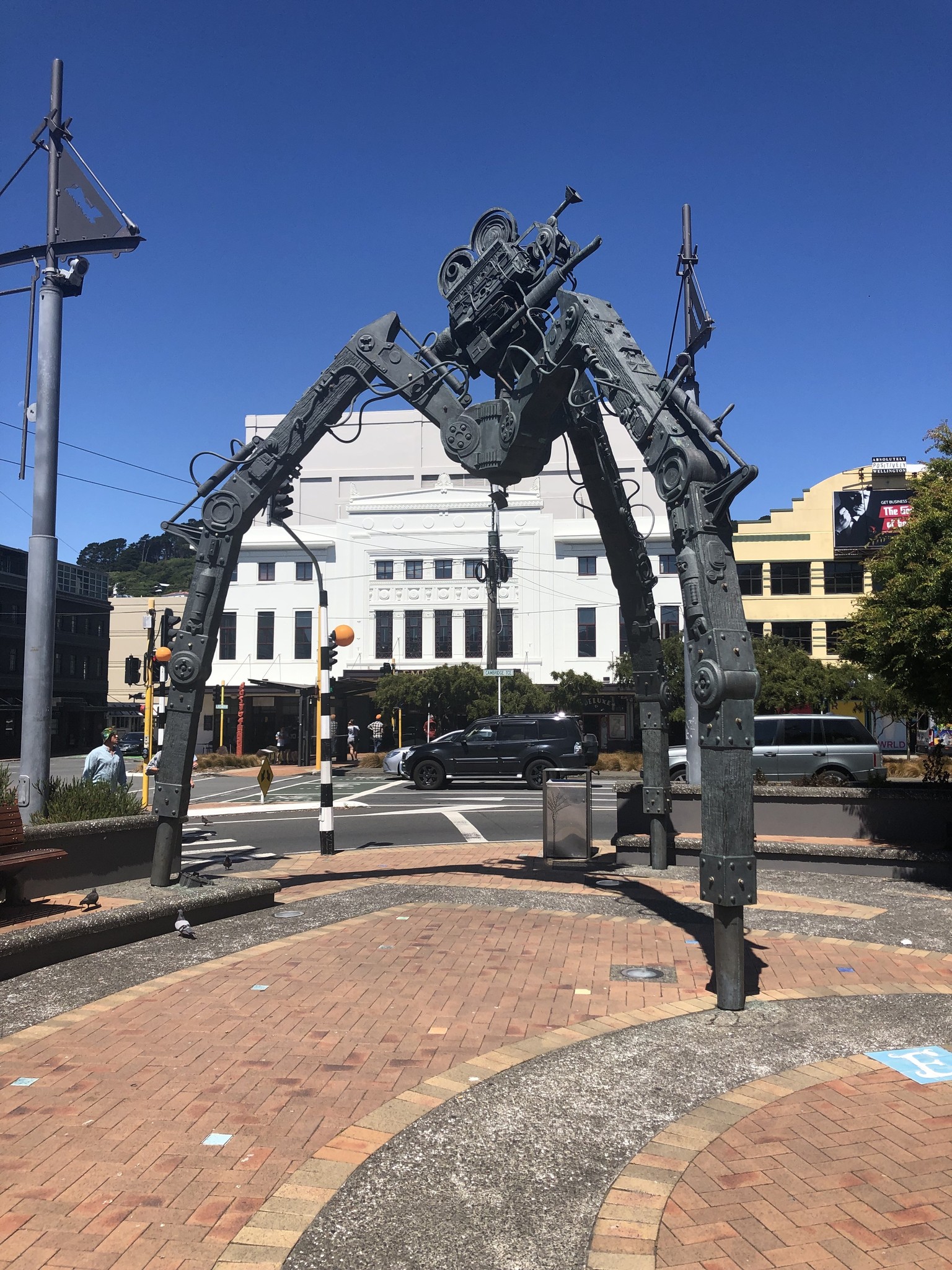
[353,727,360,743]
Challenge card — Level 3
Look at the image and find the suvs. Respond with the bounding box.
[641,713,887,789]
[404,714,601,788]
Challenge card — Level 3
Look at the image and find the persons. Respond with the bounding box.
[330,714,338,754]
[347,719,360,761]
[423,713,437,744]
[275,726,288,765]
[476,725,498,742]
[145,747,199,789]
[367,714,385,754]
[82,727,128,795]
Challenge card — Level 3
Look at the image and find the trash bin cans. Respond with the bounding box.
[541,767,594,860]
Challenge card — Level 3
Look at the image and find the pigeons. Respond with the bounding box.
[223,855,233,870]
[175,909,196,937]
[201,815,212,826]
[79,887,99,910]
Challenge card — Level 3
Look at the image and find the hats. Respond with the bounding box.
[331,714,336,720]
[101,727,117,744]
[376,714,382,720]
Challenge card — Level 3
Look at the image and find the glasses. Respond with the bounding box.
[110,733,117,737]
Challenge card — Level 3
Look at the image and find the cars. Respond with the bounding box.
[383,730,494,776]
[118,731,158,754]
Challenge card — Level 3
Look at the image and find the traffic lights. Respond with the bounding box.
[328,638,338,668]
[124,654,141,685]
[160,609,181,652]
[212,685,220,704]
